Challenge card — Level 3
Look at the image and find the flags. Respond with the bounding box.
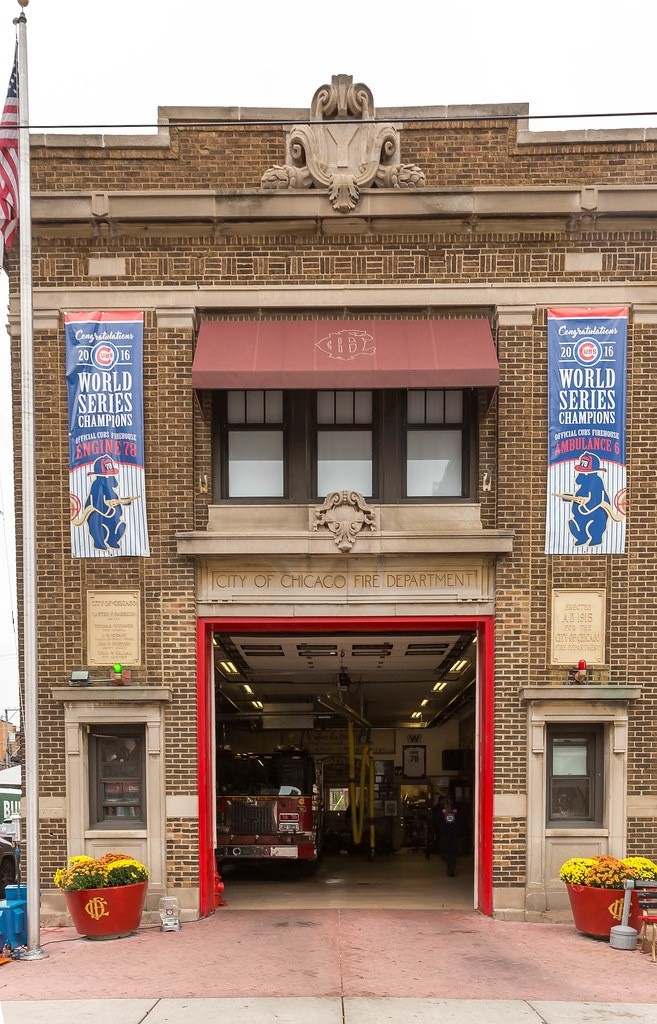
[0,34,19,250]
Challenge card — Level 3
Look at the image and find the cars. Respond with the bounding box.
[0,837,20,899]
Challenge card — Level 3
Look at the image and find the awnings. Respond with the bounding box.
[189,315,502,390]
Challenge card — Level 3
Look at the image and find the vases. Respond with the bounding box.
[64,879,148,941]
[565,883,643,940]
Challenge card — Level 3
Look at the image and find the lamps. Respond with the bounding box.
[69,670,93,687]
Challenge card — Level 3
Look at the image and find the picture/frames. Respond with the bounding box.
[384,800,398,817]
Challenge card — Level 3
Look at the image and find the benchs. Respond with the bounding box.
[635,879,657,963]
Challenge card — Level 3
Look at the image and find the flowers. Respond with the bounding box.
[559,854,657,888]
[53,853,150,895]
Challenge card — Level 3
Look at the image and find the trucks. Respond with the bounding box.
[214,744,325,878]
